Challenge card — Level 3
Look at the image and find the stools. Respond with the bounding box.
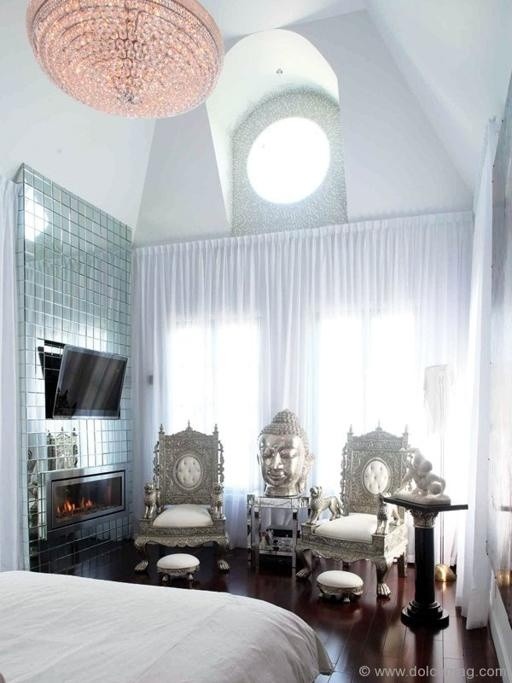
[317,569,363,602]
[157,554,201,581]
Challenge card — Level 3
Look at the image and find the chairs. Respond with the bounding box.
[295,422,414,599]
[131,420,231,573]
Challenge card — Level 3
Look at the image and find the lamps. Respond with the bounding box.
[25,0,224,119]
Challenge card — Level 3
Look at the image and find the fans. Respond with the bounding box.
[246,495,312,574]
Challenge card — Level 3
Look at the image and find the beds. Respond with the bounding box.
[0,569,335,683]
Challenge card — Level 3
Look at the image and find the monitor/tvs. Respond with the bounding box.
[52,343,129,421]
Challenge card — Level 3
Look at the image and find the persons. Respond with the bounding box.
[256,409,310,499]
